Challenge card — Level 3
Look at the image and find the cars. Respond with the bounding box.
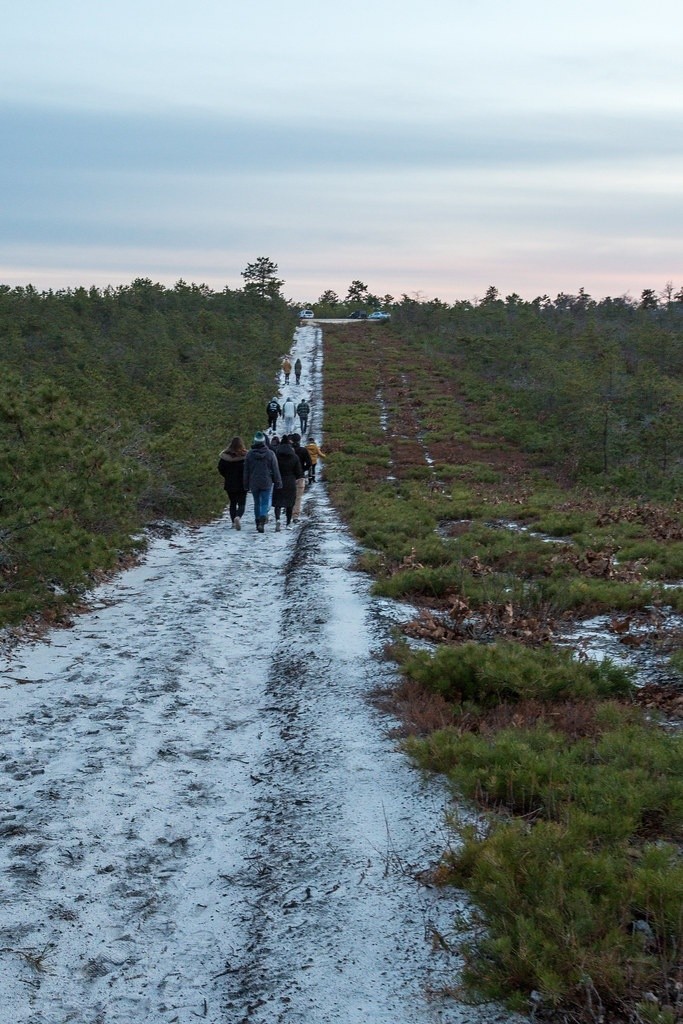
[368,311,393,319]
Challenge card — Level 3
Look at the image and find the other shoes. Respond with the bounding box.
[293,519,300,523]
[275,522,282,532]
[235,517,241,530]
[286,525,292,530]
[231,523,236,528]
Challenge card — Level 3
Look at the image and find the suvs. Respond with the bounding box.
[346,310,366,319]
[298,309,314,320]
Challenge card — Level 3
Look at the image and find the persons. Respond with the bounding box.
[282,396,297,435]
[266,397,282,435]
[282,358,292,385]
[216,430,326,534]
[297,398,310,433]
[294,358,302,385]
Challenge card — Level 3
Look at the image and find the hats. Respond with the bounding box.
[253,431,266,445]
[280,436,294,444]
[292,433,301,442]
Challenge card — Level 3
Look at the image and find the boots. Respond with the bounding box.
[256,519,260,530]
[257,517,265,532]
